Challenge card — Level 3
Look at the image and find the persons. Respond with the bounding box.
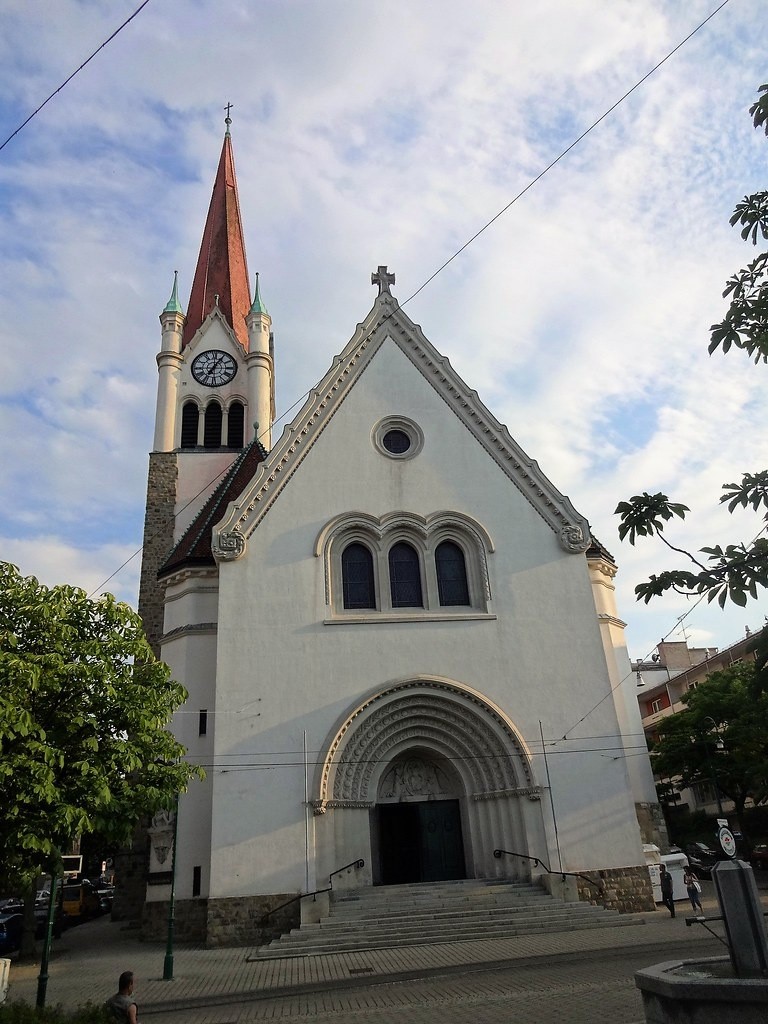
[659,864,675,918]
[683,866,705,917]
[106,971,145,1024]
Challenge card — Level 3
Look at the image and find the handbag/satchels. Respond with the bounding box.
[691,874,701,893]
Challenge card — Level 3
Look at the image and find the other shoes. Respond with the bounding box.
[694,913,698,916]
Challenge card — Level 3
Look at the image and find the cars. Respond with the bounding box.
[683,831,768,881]
[0,875,112,959]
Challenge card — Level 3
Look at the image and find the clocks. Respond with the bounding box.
[191,350,238,387]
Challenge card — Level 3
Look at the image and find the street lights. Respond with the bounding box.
[700,713,728,816]
